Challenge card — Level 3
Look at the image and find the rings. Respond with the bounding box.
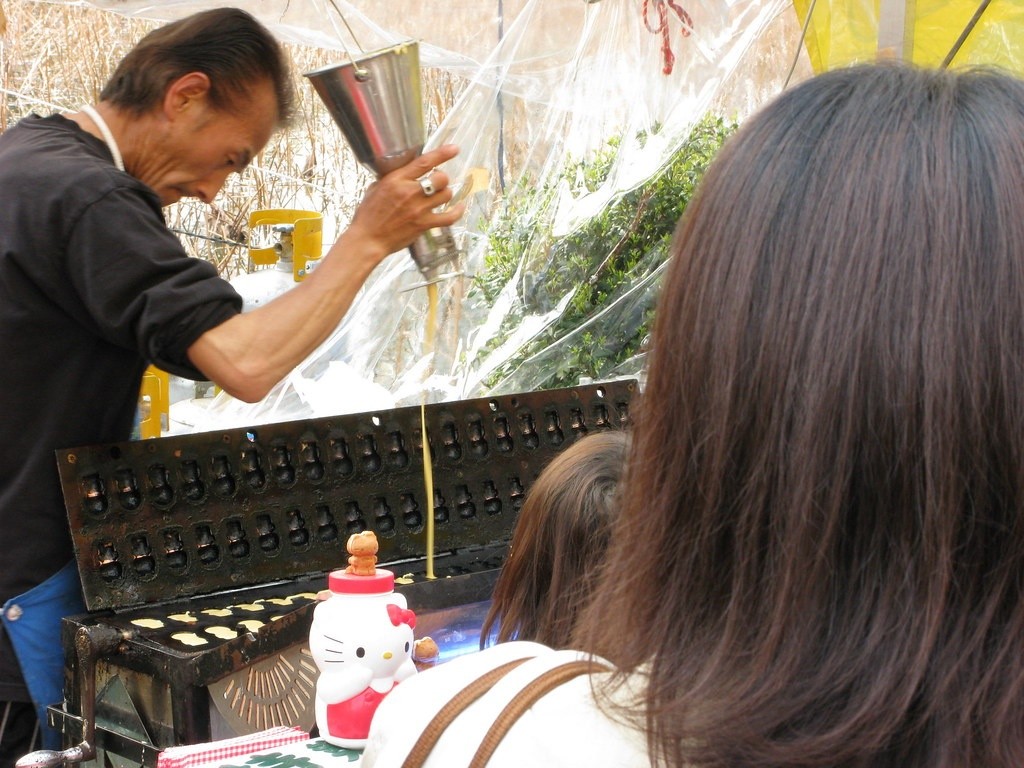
[419,176,435,197]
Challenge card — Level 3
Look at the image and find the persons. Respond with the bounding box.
[0,8,465,768]
[354,62,1024,768]
